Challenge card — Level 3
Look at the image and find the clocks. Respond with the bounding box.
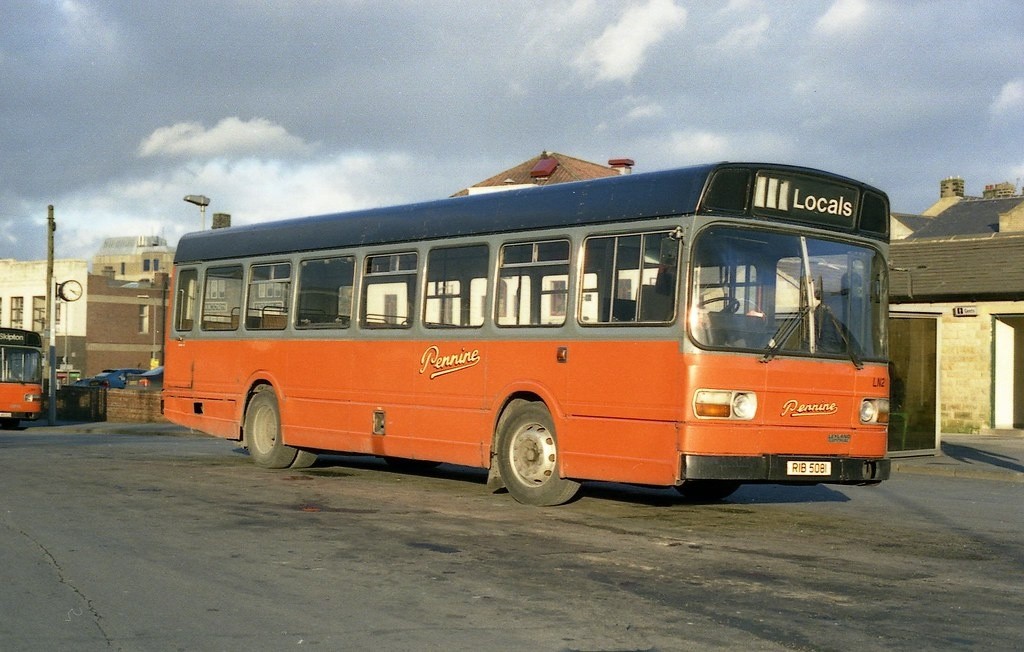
[62,282,81,300]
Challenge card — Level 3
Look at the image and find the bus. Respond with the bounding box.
[160,161,914,508]
[0,327,45,431]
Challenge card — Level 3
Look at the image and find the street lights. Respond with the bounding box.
[182,194,211,230]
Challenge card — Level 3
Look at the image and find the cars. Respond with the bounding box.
[70,366,164,393]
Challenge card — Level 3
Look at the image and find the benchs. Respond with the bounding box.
[203,313,231,330]
[260,306,326,330]
[230,305,287,329]
